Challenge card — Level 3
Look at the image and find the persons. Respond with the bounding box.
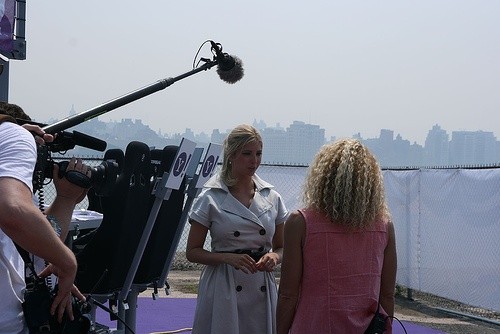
[181,122,292,333]
[0,101,95,295]
[0,107,88,334]
[273,136,397,334]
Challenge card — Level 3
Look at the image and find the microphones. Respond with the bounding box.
[62,130,107,152]
[211,39,244,83]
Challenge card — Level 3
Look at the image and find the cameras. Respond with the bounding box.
[21,276,91,334]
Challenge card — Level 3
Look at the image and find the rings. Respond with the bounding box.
[267,260,271,265]
